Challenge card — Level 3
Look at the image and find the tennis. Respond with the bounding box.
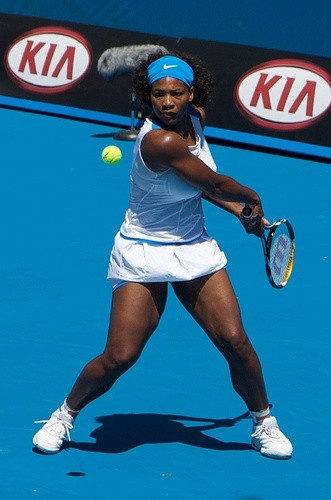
[101,145,122,165]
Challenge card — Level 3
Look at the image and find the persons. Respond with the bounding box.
[32,49,295,461]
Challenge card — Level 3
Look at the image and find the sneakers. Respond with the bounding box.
[252,416,294,459]
[33,408,74,454]
